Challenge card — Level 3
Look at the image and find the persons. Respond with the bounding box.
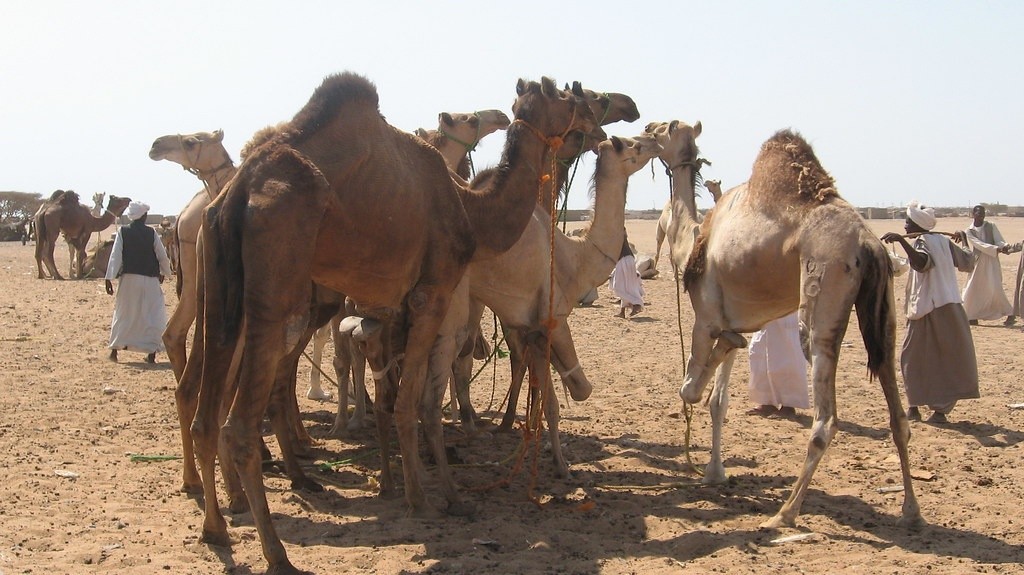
[103,201,171,364]
[880,202,980,422]
[746,310,811,420]
[1001,240,1024,325]
[161,215,171,227]
[960,205,1015,326]
[608,226,644,319]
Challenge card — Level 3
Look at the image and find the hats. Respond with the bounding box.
[127,201,150,220]
[906,200,937,230]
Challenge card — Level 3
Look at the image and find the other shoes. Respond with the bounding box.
[926,414,947,423]
[906,407,921,420]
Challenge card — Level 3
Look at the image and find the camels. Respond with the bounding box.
[145,68,660,574]
[645,118,928,531]
[34,189,131,281]
